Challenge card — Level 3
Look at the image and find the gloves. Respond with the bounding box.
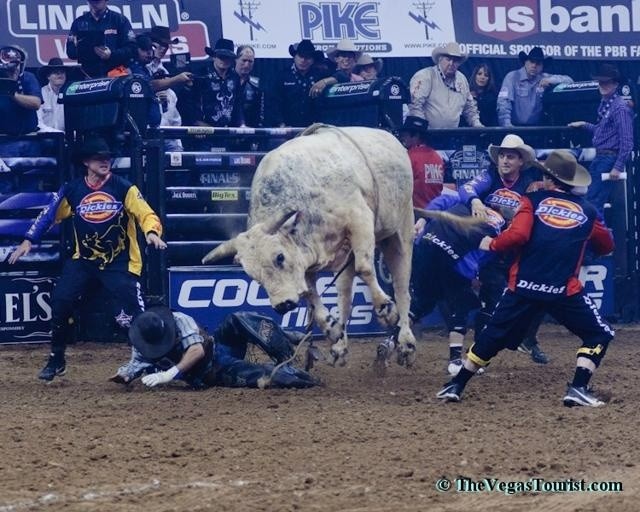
[141,365,181,388]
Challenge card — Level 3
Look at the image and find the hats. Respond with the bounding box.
[591,63,622,83]
[204,38,243,61]
[323,39,365,64]
[77,136,117,162]
[125,303,179,361]
[396,114,431,137]
[134,25,181,47]
[288,39,324,61]
[518,45,554,69]
[486,133,537,172]
[533,148,593,188]
[430,41,467,69]
[352,52,385,77]
[37,56,83,81]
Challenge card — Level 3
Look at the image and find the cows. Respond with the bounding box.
[200,123,491,375]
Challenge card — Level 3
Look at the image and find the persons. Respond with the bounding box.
[1,2,639,407]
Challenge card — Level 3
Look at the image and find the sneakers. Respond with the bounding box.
[371,337,396,378]
[516,340,550,365]
[38,357,67,384]
[561,382,607,408]
[447,358,487,378]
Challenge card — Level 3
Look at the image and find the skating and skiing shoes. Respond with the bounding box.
[434,380,465,402]
